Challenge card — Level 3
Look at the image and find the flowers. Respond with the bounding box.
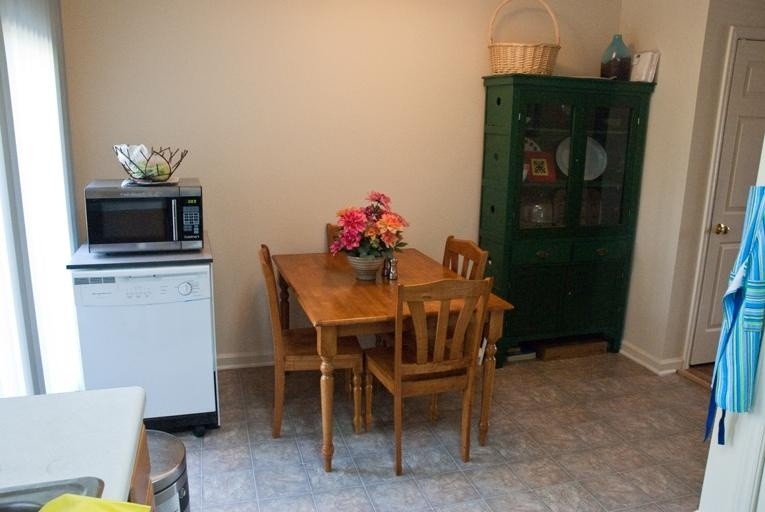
[328,189,407,258]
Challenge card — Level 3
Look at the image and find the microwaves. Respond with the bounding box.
[81,176,209,255]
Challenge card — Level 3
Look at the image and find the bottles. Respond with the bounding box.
[382,253,399,282]
[598,32,633,81]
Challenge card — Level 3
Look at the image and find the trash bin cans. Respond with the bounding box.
[144,429,189,512]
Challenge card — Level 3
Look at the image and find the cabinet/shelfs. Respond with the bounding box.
[477,74,658,368]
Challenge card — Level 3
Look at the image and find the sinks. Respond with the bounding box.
[0,478,102,512]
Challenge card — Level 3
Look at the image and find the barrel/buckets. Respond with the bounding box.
[601,33,631,79]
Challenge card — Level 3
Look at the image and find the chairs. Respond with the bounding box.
[257,235,494,475]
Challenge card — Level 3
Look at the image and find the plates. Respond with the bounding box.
[555,134,608,182]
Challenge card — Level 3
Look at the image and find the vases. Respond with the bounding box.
[344,253,385,283]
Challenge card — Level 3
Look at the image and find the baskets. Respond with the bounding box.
[486,0,561,77]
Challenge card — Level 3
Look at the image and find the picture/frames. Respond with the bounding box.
[524,149,556,183]
